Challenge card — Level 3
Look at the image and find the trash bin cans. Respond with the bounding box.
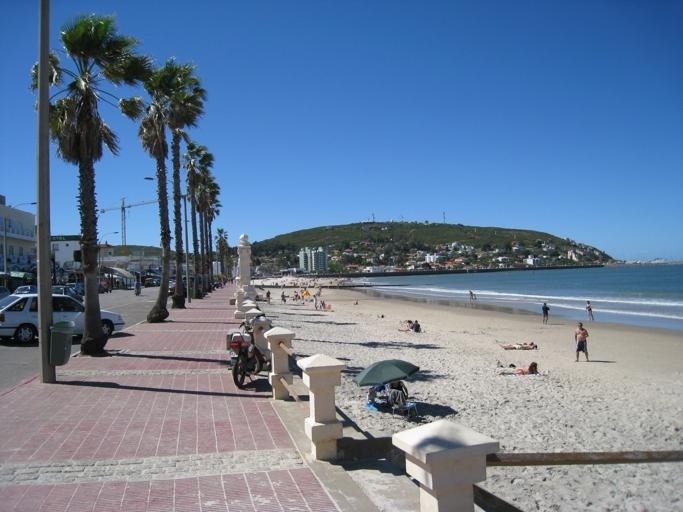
[50,320,76,366]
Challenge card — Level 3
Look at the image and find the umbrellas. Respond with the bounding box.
[351,359,420,394]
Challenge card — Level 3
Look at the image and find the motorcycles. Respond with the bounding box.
[230,313,265,388]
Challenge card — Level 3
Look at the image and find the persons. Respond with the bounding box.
[280,292,286,304]
[499,344,538,350]
[367,387,376,405]
[134,279,140,296]
[410,319,421,333]
[388,379,408,400]
[498,362,540,375]
[573,322,589,362]
[292,290,298,302]
[397,319,414,332]
[299,285,326,311]
[265,290,271,304]
[507,342,534,346]
[540,302,549,325]
[468,290,473,302]
[585,301,593,321]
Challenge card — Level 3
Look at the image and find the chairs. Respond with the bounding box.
[390,389,418,417]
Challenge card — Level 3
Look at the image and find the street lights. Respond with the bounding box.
[98,232,119,283]
[4,202,37,290]
[144,177,191,303]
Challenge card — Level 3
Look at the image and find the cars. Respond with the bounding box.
[0,280,125,343]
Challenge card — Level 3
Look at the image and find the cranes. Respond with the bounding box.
[100,196,159,246]
